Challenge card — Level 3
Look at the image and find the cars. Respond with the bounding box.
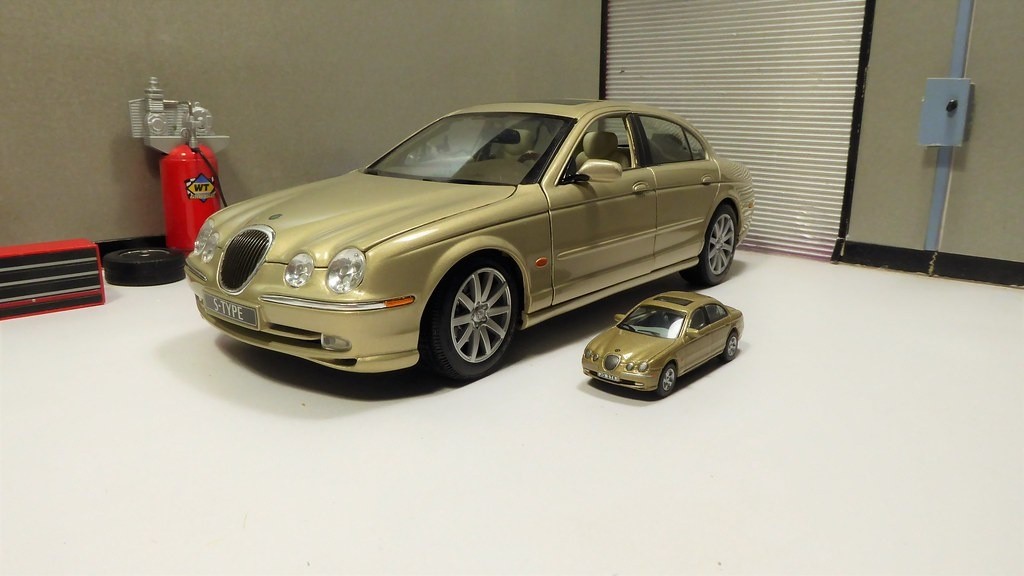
[180,98,753,383]
[580,290,744,399]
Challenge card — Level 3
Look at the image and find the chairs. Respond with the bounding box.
[476,131,682,175]
[650,307,716,329]
